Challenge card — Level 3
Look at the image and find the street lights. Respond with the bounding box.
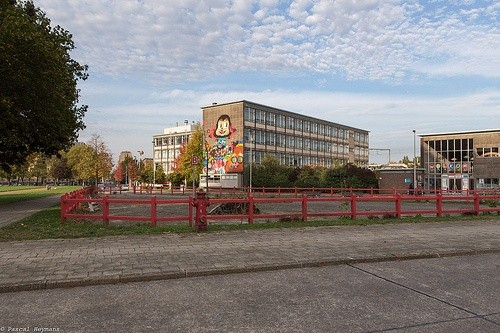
[412,129,419,189]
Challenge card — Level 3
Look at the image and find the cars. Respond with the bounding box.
[121,184,129,191]
